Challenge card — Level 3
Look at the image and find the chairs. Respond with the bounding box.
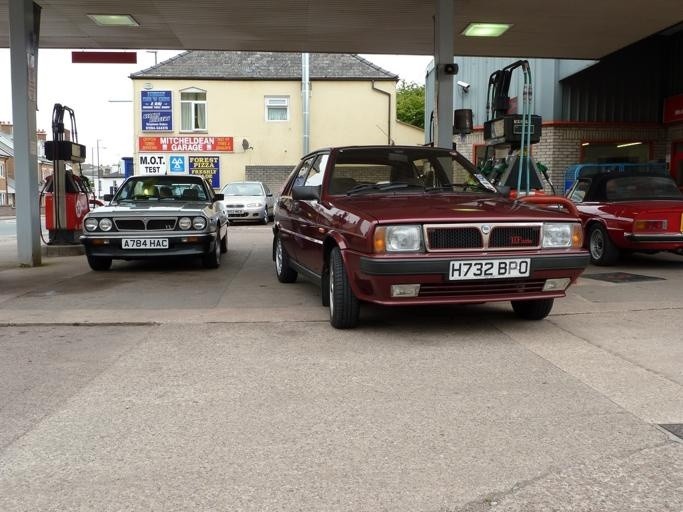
[332,178,356,194]
[183,189,198,197]
[390,161,413,184]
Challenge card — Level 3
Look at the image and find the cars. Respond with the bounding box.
[219,182,275,224]
[566,172,683,266]
[273,146,591,329]
[79,175,229,271]
